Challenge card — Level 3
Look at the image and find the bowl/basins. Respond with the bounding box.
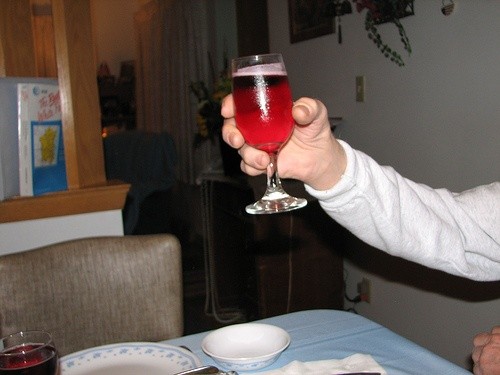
[200,321,291,372]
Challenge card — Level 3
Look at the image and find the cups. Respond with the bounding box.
[0,329,57,375]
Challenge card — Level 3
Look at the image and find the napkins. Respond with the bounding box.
[251,352,389,375]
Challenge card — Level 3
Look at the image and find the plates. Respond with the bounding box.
[57,341,200,375]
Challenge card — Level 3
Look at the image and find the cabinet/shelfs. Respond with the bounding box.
[219,117,349,320]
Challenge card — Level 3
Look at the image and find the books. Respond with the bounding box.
[17,83,68,197]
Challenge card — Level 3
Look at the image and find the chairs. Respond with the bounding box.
[105,129,193,250]
[0,234,185,357]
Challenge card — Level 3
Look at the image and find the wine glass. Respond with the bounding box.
[230,51,308,215]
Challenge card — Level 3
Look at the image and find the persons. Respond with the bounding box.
[221,92,500,374]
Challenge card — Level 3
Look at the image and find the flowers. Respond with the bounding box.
[189,49,233,154]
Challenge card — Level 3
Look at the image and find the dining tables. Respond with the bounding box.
[157,310,480,375]
